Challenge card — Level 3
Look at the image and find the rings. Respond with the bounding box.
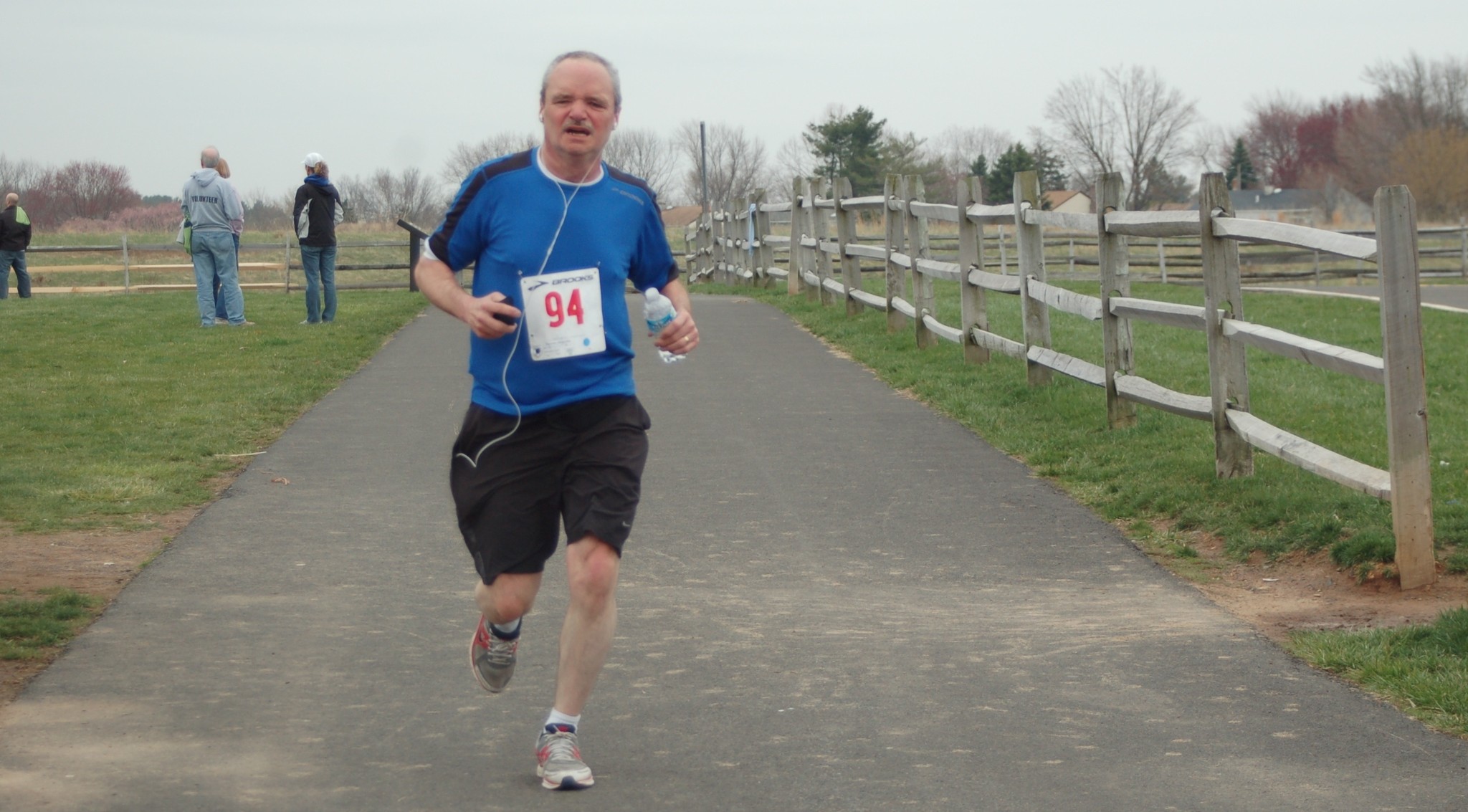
[684,336,690,344]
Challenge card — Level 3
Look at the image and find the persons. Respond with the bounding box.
[292,153,344,325]
[413,50,701,792]
[181,145,255,328]
[0,193,31,299]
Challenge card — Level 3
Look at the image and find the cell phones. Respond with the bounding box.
[494,294,516,325]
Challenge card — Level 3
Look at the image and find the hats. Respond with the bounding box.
[301,153,324,167]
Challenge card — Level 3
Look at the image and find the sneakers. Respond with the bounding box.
[535,723,595,790]
[469,614,522,693]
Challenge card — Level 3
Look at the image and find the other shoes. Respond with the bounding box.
[299,320,323,324]
[240,321,255,326]
[215,319,229,325]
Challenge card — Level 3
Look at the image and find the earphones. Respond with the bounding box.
[613,116,618,130]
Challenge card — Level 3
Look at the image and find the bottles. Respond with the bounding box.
[643,287,688,364]
